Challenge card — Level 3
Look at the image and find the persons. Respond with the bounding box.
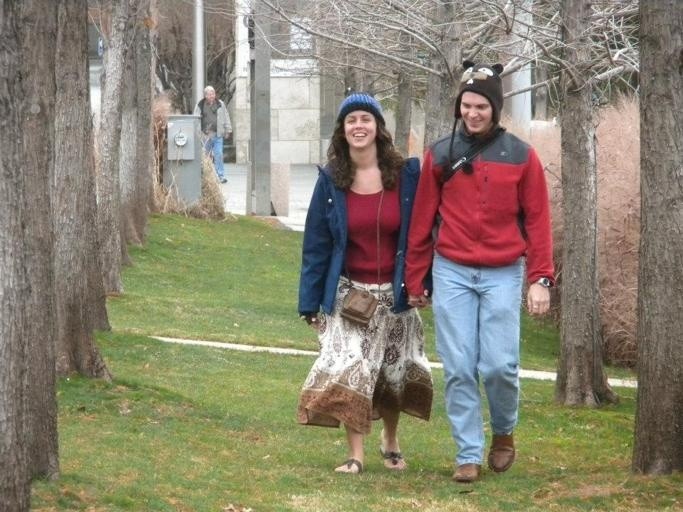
[404,60,555,481]
[297,94,434,475]
[194,85,232,183]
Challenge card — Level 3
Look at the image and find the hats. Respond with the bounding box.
[334,91,383,123]
[451,57,504,123]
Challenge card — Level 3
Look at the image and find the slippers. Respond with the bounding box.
[379,443,407,471]
[332,455,364,475]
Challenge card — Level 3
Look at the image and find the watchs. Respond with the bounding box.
[537,277,553,287]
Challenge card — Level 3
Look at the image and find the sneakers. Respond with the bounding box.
[219,174,227,184]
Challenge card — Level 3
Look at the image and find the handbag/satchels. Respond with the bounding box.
[339,287,379,327]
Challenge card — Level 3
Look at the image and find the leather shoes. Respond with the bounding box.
[452,462,483,484]
[487,431,517,474]
